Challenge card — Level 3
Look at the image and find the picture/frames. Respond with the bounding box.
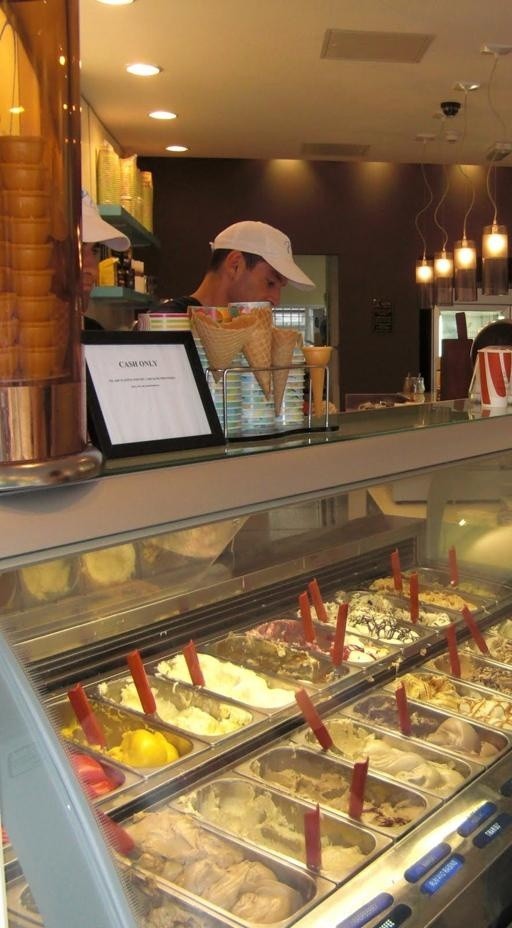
[81,329,227,462]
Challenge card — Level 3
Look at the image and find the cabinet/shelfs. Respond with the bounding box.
[2,397,512,928]
[84,203,157,330]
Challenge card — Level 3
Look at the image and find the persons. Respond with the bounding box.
[148,221,315,314]
[81,190,131,333]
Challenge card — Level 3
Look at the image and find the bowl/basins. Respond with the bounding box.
[138,301,305,432]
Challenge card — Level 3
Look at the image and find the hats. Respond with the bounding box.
[81,187,131,254]
[209,221,316,292]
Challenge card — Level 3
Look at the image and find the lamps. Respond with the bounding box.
[414,135,431,310]
[454,80,479,302]
[480,43,512,298]
[432,100,455,306]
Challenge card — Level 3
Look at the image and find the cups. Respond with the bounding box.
[477,349,511,408]
[98,148,153,233]
